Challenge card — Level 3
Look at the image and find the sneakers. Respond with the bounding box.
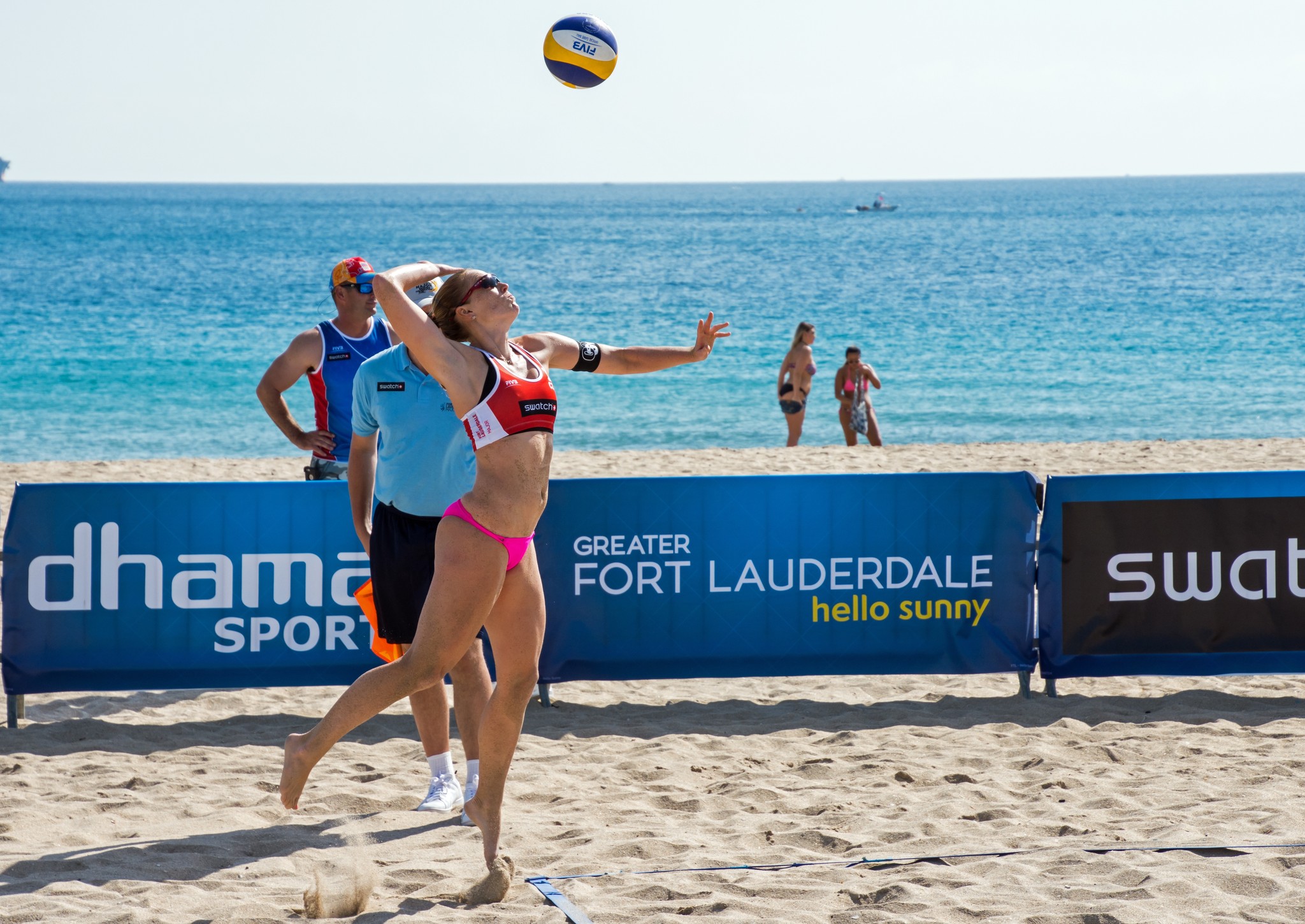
[461,775,479,826]
[417,772,462,814]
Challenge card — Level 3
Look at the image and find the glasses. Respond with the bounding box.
[448,274,500,321]
[341,283,373,294]
[431,315,440,329]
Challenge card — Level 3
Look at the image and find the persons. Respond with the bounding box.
[776,321,816,448]
[834,346,882,448]
[255,257,446,484]
[345,261,492,830]
[280,258,729,881]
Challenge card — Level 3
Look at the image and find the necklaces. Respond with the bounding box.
[490,352,517,364]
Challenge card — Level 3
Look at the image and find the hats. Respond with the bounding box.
[404,277,444,309]
[328,257,378,292]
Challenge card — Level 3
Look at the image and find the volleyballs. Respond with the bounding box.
[544,14,619,89]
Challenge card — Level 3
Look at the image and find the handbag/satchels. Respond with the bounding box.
[849,369,868,436]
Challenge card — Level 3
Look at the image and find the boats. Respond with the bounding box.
[859,204,897,212]
